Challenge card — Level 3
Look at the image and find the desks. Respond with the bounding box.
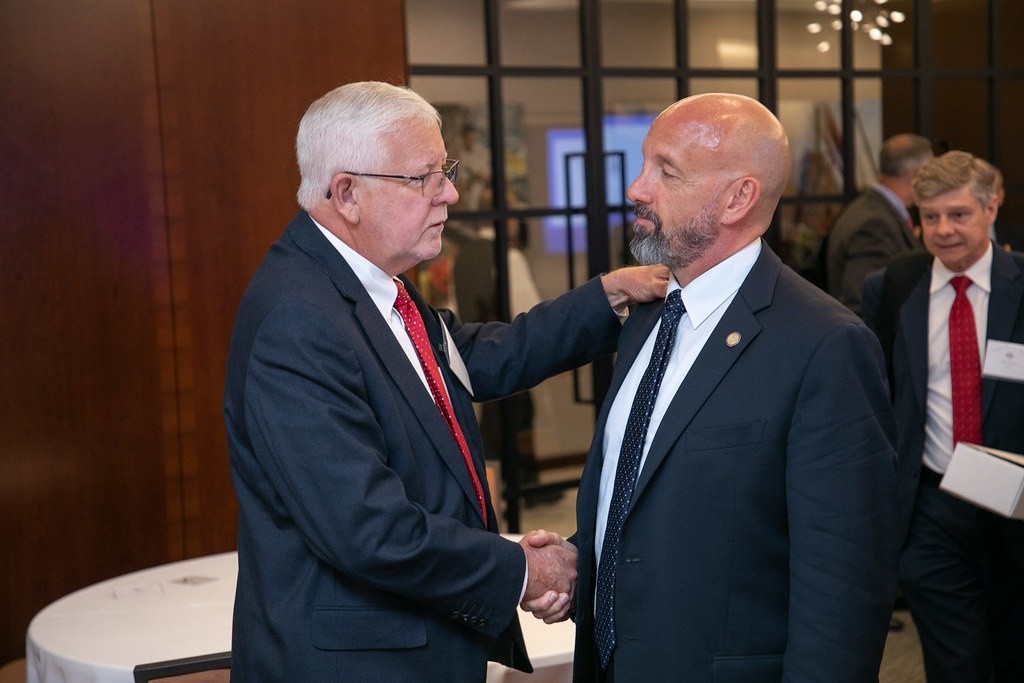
[25,531,575,683]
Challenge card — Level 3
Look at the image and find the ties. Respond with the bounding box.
[948,275,983,448]
[393,278,487,529]
[595,289,686,670]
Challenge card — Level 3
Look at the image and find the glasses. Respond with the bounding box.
[326,158,460,199]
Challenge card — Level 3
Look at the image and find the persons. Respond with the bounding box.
[520,93,899,683]
[827,133,934,632]
[451,124,491,209]
[222,81,669,683]
[453,188,565,510]
[860,150,1024,683]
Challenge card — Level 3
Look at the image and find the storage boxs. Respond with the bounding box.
[940,440,1024,524]
